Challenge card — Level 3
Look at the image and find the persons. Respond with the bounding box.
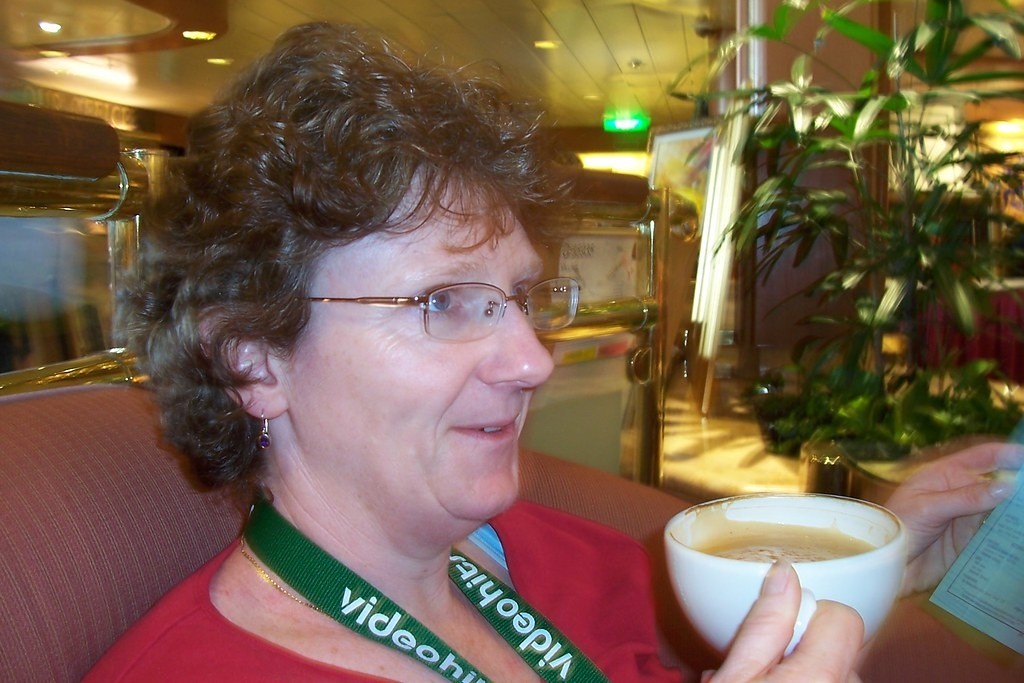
[79,22,1024,683]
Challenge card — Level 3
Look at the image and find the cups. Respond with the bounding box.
[663,491,906,661]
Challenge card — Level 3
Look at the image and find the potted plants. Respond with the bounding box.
[661,1,1024,504]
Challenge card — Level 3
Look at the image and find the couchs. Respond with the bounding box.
[0,384,1022,682]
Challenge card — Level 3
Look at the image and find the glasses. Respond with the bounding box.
[301,276,580,344]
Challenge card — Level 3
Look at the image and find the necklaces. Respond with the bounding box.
[240,538,330,619]
